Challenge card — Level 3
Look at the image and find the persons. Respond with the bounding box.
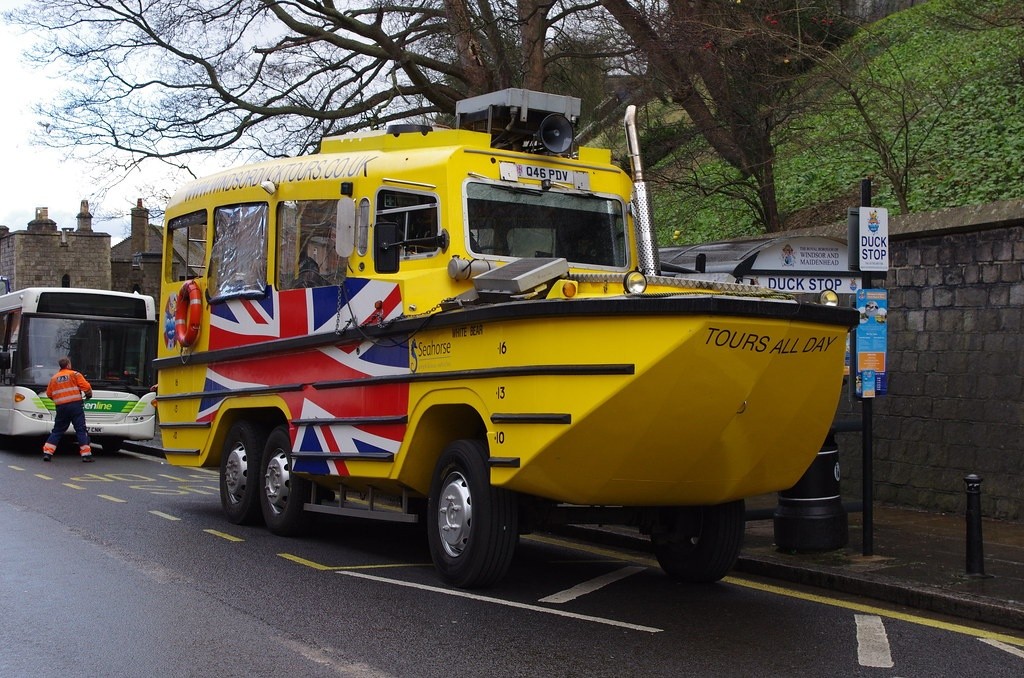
[289,257,332,291]
[42,358,96,464]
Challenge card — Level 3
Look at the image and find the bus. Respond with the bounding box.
[0,284,157,454]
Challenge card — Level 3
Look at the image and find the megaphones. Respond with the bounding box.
[533,113,574,154]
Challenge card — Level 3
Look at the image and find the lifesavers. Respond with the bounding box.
[174,280,202,348]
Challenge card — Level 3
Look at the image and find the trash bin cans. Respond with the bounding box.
[779,424,840,504]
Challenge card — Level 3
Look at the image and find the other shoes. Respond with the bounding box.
[83,456,96,462]
[43,454,51,460]
